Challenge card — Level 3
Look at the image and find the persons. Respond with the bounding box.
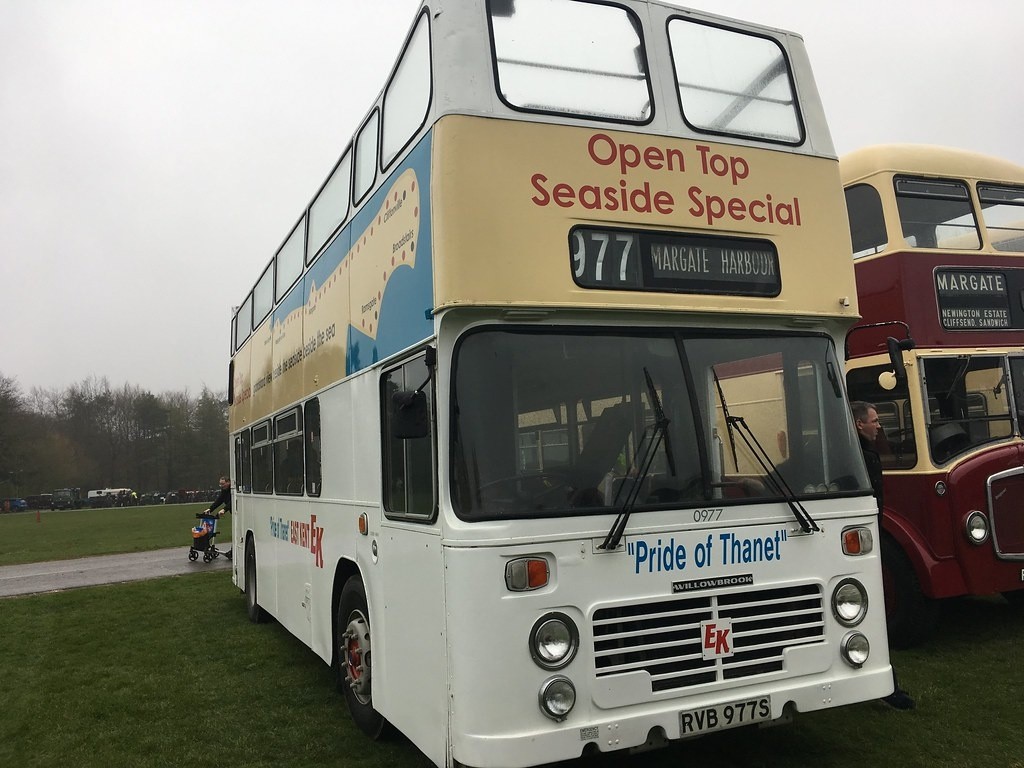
[131,490,138,506]
[204,475,233,560]
[850,400,883,523]
[740,442,826,495]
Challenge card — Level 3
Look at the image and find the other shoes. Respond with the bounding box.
[224,550,232,557]
[885,689,916,710]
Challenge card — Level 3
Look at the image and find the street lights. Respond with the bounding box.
[10,468,23,501]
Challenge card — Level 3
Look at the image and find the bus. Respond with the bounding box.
[87,488,133,507]
[208,0,919,768]
[517,143,1024,638]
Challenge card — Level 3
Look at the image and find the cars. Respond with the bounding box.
[139,488,221,505]
[0,493,52,514]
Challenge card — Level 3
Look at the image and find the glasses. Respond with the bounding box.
[219,483,229,487]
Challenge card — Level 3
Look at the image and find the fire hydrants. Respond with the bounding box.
[34,510,41,523]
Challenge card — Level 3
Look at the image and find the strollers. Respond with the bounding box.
[188,511,220,564]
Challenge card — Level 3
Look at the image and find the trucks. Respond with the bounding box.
[49,488,84,510]
